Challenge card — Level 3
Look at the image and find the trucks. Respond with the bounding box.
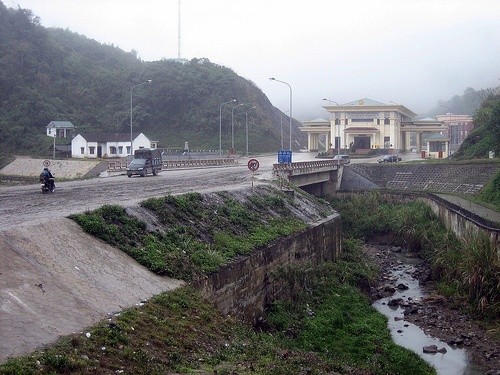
[126,148,163,177]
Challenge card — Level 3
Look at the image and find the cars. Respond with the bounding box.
[376,155,402,163]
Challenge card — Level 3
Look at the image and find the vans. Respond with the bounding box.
[332,155,350,165]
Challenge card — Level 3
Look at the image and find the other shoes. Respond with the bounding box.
[52,189,54,193]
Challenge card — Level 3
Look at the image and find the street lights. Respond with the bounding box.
[219,99,237,159]
[232,103,243,149]
[281,110,290,151]
[322,98,340,160]
[246,106,256,157]
[268,77,292,152]
[130,80,152,156]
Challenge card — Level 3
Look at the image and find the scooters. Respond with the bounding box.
[41,173,56,194]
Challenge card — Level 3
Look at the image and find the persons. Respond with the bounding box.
[40,167,55,191]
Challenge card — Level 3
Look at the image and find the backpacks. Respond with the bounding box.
[39,172,48,182]
